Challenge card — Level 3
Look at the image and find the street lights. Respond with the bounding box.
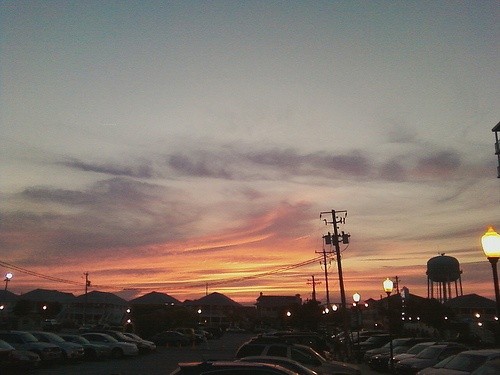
[481,226,500,308]
[382,277,394,375]
[352,291,361,363]
[332,304,337,334]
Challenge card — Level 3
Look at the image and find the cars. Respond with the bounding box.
[0,321,499,375]
